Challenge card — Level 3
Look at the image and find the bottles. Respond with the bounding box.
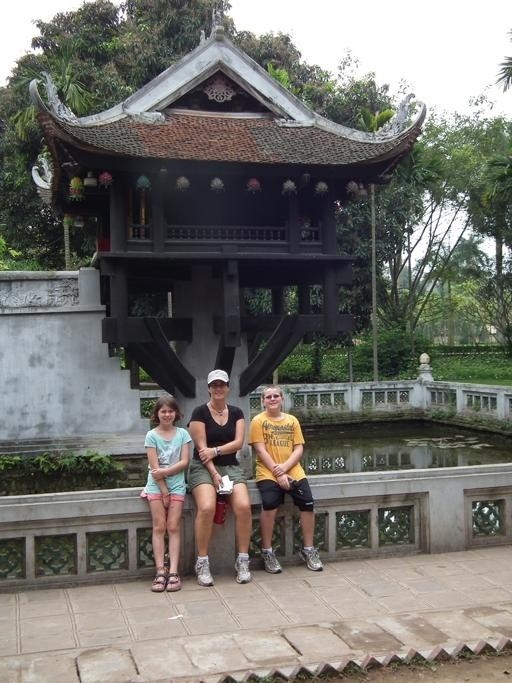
[213,496,226,524]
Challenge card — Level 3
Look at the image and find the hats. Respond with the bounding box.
[207,369,229,385]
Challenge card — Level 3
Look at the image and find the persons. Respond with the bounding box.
[186,369,253,586]
[246,383,325,572]
[139,395,192,592]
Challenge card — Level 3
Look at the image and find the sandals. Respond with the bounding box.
[151,570,182,592]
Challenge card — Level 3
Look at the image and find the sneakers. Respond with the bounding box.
[234,555,251,584]
[261,547,282,574]
[299,546,323,571]
[195,557,214,587]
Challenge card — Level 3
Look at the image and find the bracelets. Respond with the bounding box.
[213,447,218,456]
[212,471,219,479]
[216,446,222,456]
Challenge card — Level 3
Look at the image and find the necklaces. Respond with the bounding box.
[210,399,226,417]
